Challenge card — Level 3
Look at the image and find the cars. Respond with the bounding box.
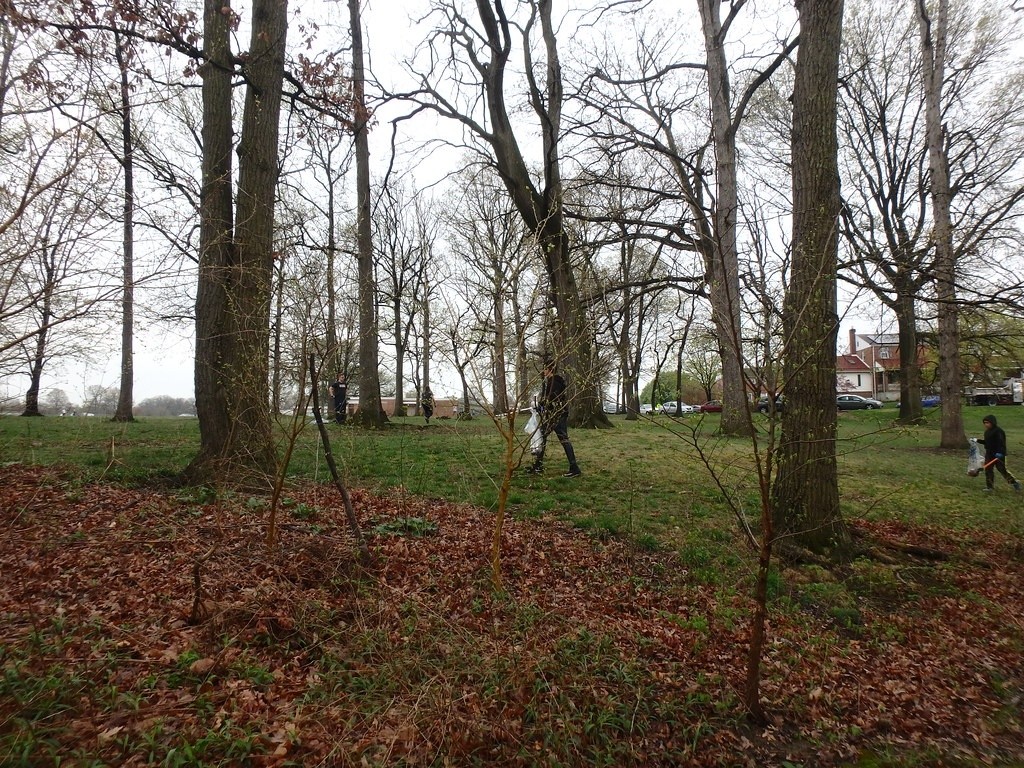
[757,398,782,414]
[920,395,941,406]
[836,394,884,412]
[603,401,722,415]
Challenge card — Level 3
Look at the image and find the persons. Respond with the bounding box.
[972,414,1020,493]
[421,385,437,424]
[329,373,349,424]
[452,403,458,415]
[525,359,582,477]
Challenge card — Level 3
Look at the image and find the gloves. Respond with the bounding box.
[996,453,1003,459]
[971,438,979,443]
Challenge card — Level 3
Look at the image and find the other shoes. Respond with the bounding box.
[1015,480,1022,493]
[562,471,580,478]
[982,488,994,492]
[425,417,429,423]
[526,467,542,473]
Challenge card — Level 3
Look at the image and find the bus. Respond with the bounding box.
[970,387,1023,406]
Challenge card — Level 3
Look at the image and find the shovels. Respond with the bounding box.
[968,458,998,477]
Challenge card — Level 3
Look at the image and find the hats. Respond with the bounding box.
[545,359,557,374]
